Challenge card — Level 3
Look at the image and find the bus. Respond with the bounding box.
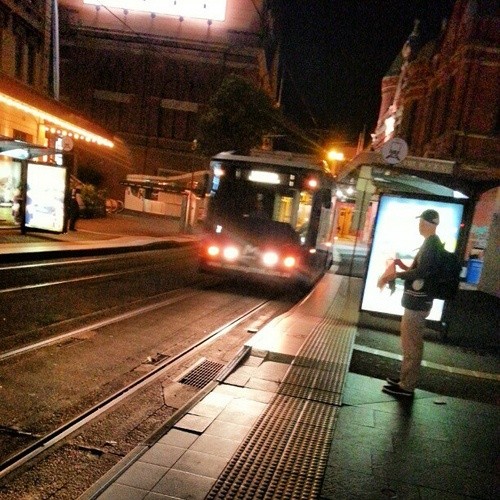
[198,132,343,294]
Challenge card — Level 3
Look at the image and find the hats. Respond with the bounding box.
[415,209,439,225]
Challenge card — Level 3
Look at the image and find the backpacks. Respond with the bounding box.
[425,252,460,299]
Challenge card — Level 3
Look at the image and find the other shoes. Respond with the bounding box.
[382,385,415,397]
[386,376,402,386]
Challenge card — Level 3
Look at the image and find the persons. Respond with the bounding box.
[381,210,444,397]
[248,199,272,221]
[69,182,84,231]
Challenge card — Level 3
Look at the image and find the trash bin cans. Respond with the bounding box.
[465,257,484,285]
